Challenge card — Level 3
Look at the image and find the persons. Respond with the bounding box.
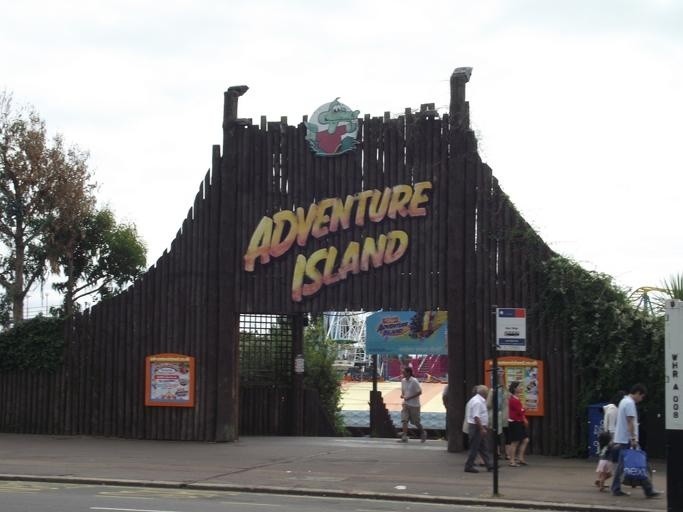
[462,381,531,473]
[397,368,427,443]
[595,384,664,498]
[443,385,447,440]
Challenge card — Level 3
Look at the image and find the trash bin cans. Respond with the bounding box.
[584,401,616,463]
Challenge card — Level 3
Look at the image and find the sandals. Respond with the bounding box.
[592,479,609,492]
[480,463,485,467]
[497,453,528,468]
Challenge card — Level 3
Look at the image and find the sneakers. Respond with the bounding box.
[612,491,631,496]
[646,491,665,497]
[421,431,427,442]
[395,437,409,442]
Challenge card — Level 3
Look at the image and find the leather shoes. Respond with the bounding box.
[488,467,493,472]
[464,467,479,473]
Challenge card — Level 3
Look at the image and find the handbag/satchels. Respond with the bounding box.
[620,447,653,488]
[597,431,615,460]
